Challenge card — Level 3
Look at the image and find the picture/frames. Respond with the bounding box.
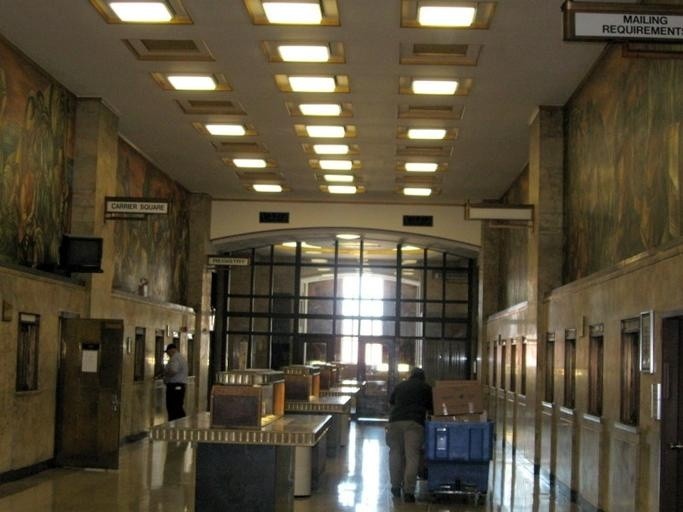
[639,309,654,374]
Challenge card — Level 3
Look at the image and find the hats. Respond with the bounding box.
[410,368,425,379]
[164,344,176,353]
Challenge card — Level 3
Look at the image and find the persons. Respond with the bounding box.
[207,358,217,411]
[385,366,434,503]
[161,344,188,421]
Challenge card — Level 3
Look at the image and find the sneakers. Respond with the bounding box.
[391,486,401,497]
[404,493,415,502]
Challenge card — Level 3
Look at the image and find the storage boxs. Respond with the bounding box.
[430,379,487,422]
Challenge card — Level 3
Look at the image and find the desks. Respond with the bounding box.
[149,378,365,510]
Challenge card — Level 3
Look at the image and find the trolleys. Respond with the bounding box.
[425,409,494,506]
